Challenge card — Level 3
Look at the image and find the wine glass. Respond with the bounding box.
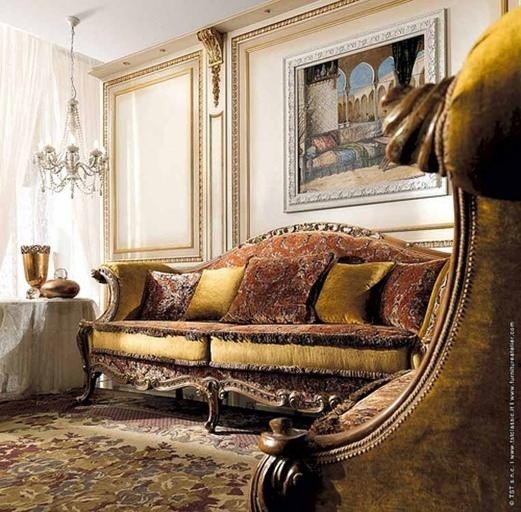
[20,244,49,298]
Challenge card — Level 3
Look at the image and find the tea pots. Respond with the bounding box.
[40,268,80,299]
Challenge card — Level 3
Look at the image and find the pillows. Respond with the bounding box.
[313,259,397,327]
[218,251,337,325]
[138,268,201,321]
[366,255,450,333]
[180,264,247,321]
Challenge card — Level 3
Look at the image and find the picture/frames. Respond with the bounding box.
[280,7,450,215]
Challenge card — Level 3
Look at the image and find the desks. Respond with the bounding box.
[0,298,101,405]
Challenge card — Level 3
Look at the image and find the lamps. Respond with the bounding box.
[32,16,107,199]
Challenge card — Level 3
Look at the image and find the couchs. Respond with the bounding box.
[246,1,519,510]
[74,221,453,435]
